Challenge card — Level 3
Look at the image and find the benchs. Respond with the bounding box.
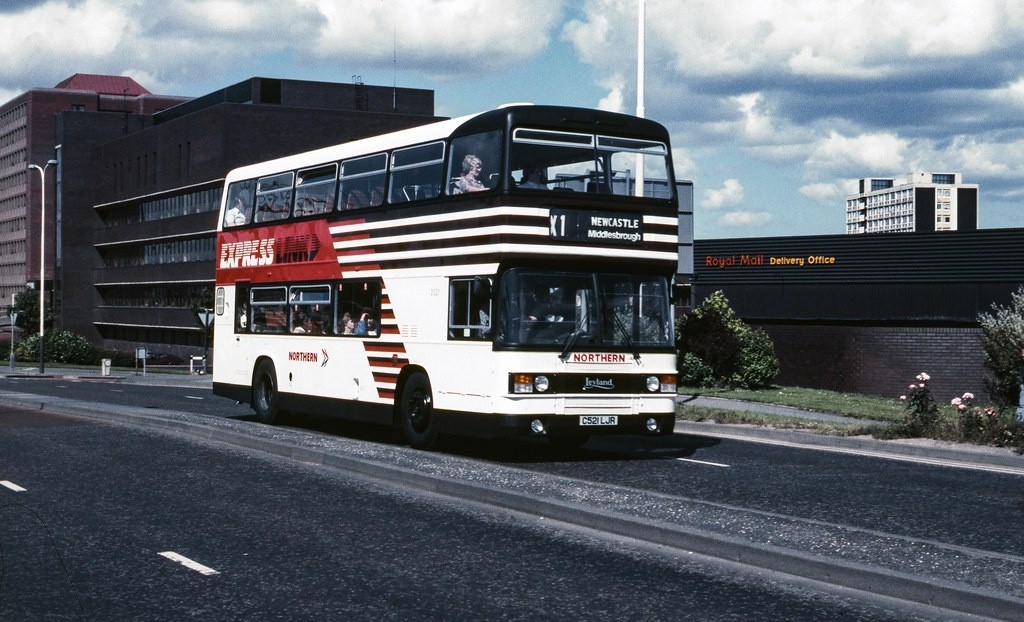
[244,175,574,225]
[241,311,359,334]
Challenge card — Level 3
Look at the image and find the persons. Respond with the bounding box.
[479,300,537,340]
[225,197,252,226]
[460,154,490,192]
[517,166,549,190]
[240,302,378,336]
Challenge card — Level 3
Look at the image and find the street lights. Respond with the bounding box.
[28,160,59,374]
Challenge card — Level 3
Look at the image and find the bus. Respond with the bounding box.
[210,101,691,450]
